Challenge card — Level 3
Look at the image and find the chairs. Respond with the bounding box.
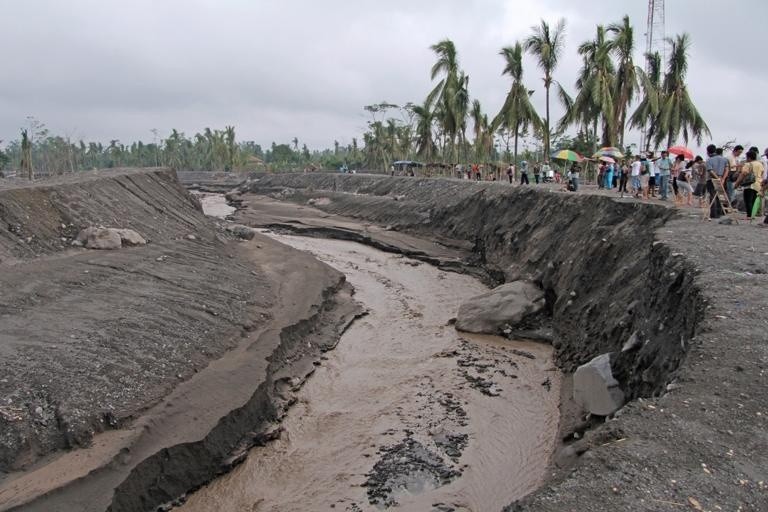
[711,178,754,225]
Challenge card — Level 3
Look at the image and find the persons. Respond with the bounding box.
[391,164,395,177]
[455,143,768,222]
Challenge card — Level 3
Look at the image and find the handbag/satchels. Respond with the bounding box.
[740,173,756,186]
[728,170,739,182]
